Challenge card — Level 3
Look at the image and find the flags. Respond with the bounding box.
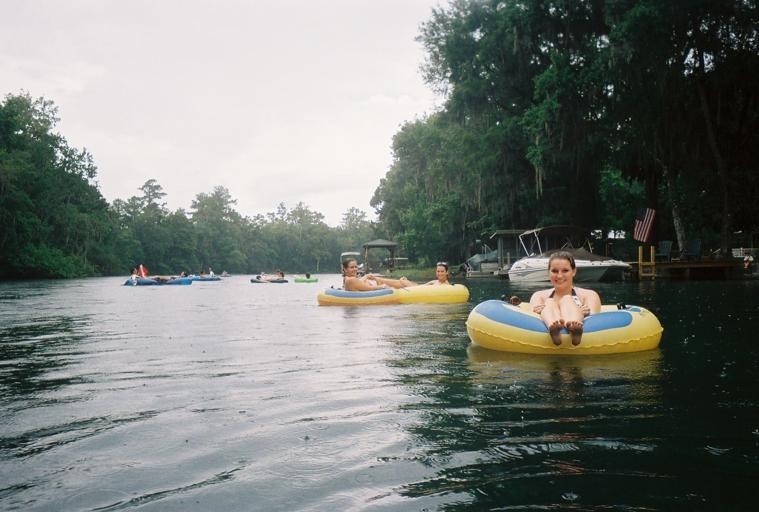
[632,208,656,242]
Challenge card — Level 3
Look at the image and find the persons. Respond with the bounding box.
[306,273,310,279]
[743,251,754,269]
[530,250,602,346]
[131,266,214,281]
[343,258,451,291]
[256,269,284,281]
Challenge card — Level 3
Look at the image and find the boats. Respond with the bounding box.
[505,224,631,286]
[461,295,664,354]
[270,278,288,284]
[398,282,471,304]
[191,274,219,282]
[462,340,663,392]
[164,277,193,284]
[123,276,158,285]
[317,286,399,305]
[339,252,365,276]
[250,278,267,283]
[294,277,319,283]
[220,274,232,277]
